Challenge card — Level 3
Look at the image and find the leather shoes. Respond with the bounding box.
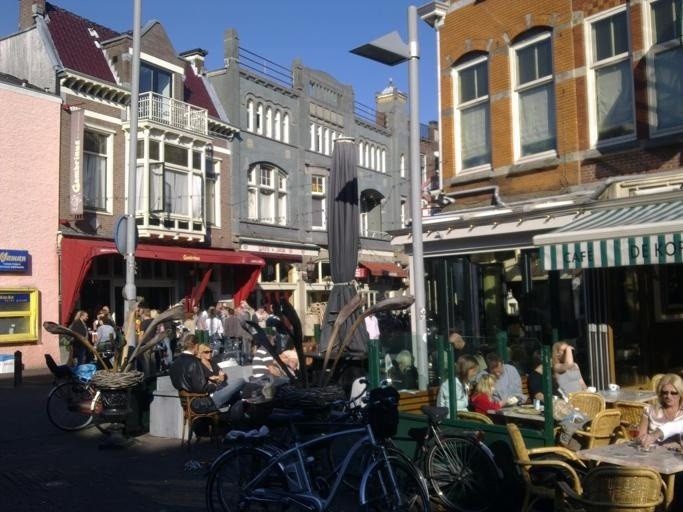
[208,448,227,462]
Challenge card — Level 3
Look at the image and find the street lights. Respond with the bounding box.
[351,6,431,387]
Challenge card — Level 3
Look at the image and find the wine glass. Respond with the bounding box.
[628,424,640,442]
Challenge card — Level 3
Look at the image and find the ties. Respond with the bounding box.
[210,318,213,334]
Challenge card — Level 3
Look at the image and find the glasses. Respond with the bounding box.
[202,350,213,354]
[661,390,679,396]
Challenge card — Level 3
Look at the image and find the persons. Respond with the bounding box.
[637,374,682,452]
[60,299,299,435]
[436,333,587,419]
[376,310,436,390]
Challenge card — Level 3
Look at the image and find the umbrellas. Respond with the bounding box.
[318,137,370,362]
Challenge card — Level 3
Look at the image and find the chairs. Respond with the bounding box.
[455,374,683,512]
[178,390,219,453]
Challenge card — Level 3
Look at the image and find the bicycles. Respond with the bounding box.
[43,354,132,433]
[207,378,505,511]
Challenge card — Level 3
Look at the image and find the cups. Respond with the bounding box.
[588,387,597,394]
[533,399,540,410]
[608,384,620,392]
